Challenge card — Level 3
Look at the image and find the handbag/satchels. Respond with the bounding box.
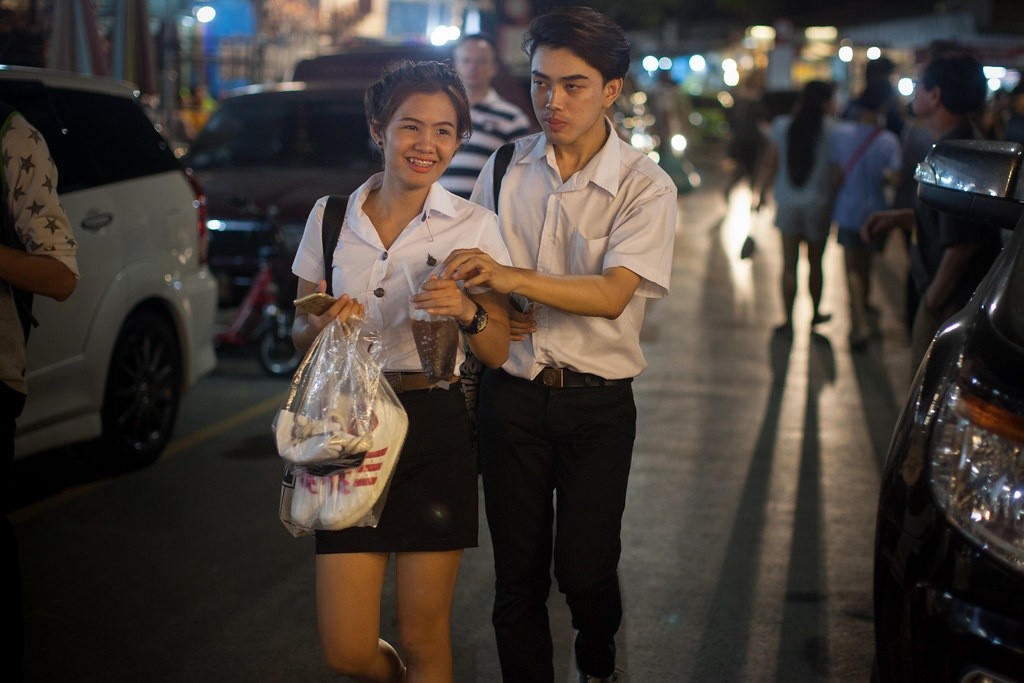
[278,320,351,539]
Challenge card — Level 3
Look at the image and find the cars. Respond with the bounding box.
[0,65,218,472]
[870,135,1024,683]
[177,80,391,311]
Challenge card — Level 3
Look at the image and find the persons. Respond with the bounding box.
[739,78,843,337]
[840,40,1004,622]
[177,85,221,143]
[468,10,678,681]
[637,51,1024,337]
[0,99,76,682]
[292,59,512,682]
[829,103,907,351]
[431,35,530,198]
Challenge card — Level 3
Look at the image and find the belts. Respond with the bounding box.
[380,373,458,393]
[536,364,633,389]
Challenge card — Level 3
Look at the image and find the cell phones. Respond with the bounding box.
[508,292,529,315]
[293,292,338,316]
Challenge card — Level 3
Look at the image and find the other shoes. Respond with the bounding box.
[811,314,831,327]
[775,324,793,338]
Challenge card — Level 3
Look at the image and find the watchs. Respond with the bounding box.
[457,294,488,335]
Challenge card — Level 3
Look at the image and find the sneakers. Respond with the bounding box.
[290,395,353,527]
[321,398,409,530]
[579,666,625,683]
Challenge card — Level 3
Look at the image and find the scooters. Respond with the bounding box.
[215,182,305,378]
